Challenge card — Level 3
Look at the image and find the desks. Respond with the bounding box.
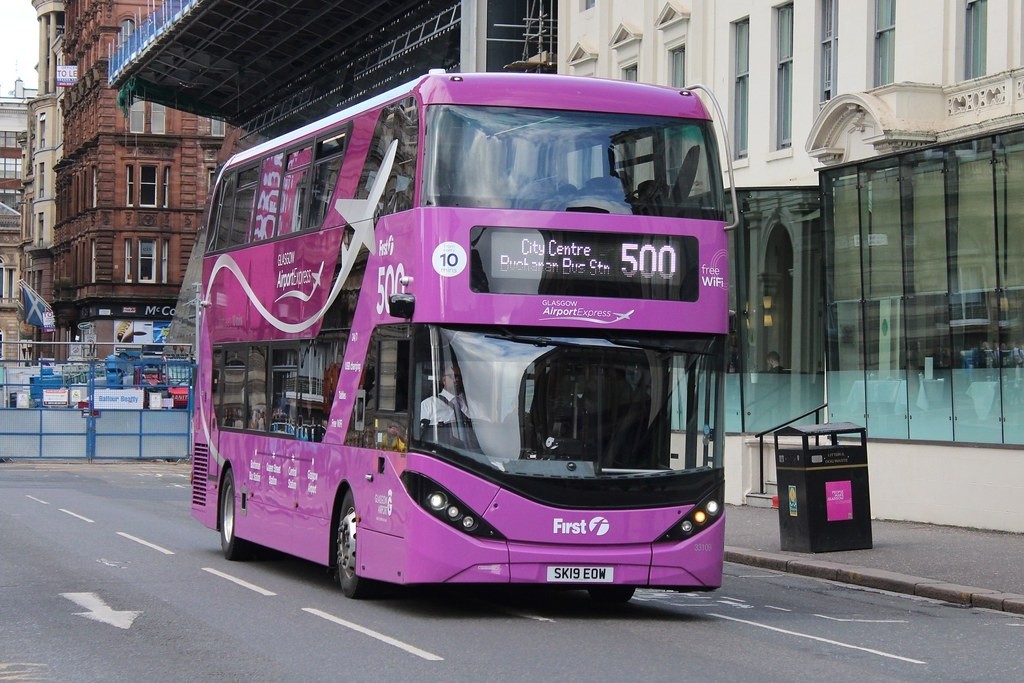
[847,379,946,411]
[966,379,1024,418]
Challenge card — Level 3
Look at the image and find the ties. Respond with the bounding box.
[455,396,462,420]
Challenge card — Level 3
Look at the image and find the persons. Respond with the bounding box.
[764,350,790,399]
[979,341,1024,368]
[416,358,470,433]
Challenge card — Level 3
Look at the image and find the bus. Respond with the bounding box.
[188,68,741,604]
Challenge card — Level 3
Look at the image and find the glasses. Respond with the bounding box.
[444,374,464,380]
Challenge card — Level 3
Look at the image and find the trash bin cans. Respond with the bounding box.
[773,422,873,552]
[10,391,17,408]
